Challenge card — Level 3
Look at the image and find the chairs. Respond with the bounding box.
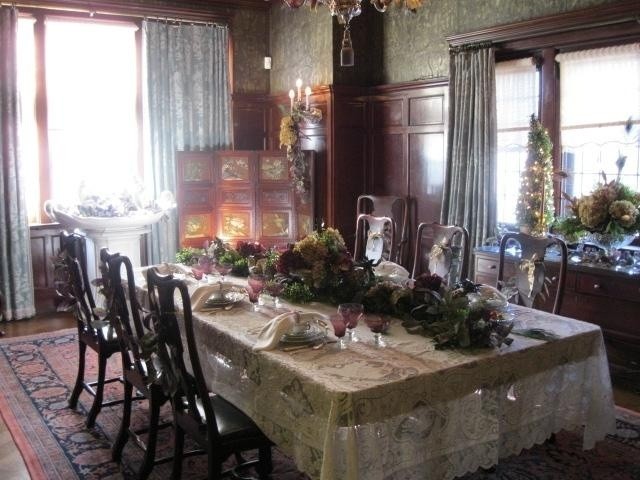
[59,230,171,428]
[99,248,248,479]
[146,267,277,478]
[353,193,567,315]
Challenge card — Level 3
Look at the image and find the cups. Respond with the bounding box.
[494,224,511,253]
[491,314,515,337]
[248,276,264,302]
[189,255,232,285]
[293,321,310,334]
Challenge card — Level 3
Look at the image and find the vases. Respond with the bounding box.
[592,228,637,259]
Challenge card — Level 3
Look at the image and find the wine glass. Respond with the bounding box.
[365,314,391,349]
[337,303,364,343]
[329,314,349,350]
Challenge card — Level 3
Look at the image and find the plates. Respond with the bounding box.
[205,292,245,305]
[279,327,324,344]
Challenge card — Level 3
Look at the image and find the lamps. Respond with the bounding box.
[278,0,423,68]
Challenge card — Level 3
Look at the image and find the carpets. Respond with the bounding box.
[1,327,638,478]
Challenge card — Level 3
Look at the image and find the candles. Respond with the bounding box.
[288,79,312,115]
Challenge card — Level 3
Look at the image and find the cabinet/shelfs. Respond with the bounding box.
[471,244,639,394]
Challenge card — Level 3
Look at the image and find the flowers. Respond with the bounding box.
[179,228,497,350]
[274,101,324,195]
[563,152,640,241]
